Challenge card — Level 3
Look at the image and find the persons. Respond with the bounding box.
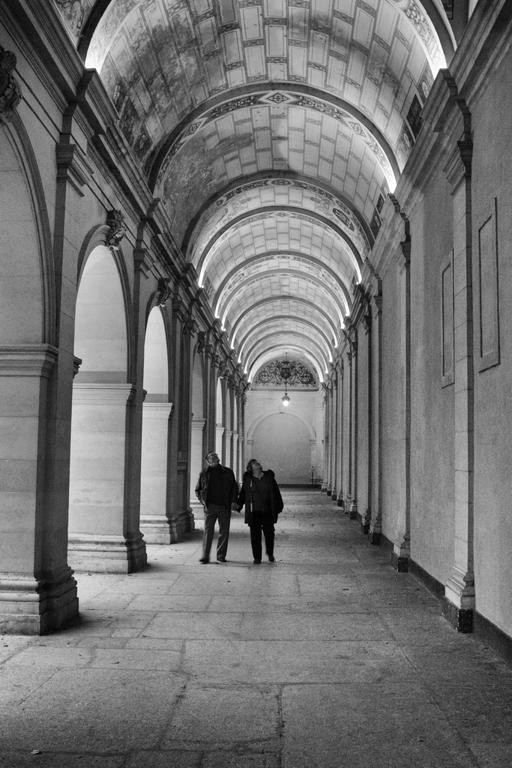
[236,458,284,564]
[194,451,239,563]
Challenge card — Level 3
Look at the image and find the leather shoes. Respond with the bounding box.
[199,557,209,562]
[217,557,227,562]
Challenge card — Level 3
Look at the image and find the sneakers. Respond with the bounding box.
[265,551,274,562]
[253,559,262,564]
